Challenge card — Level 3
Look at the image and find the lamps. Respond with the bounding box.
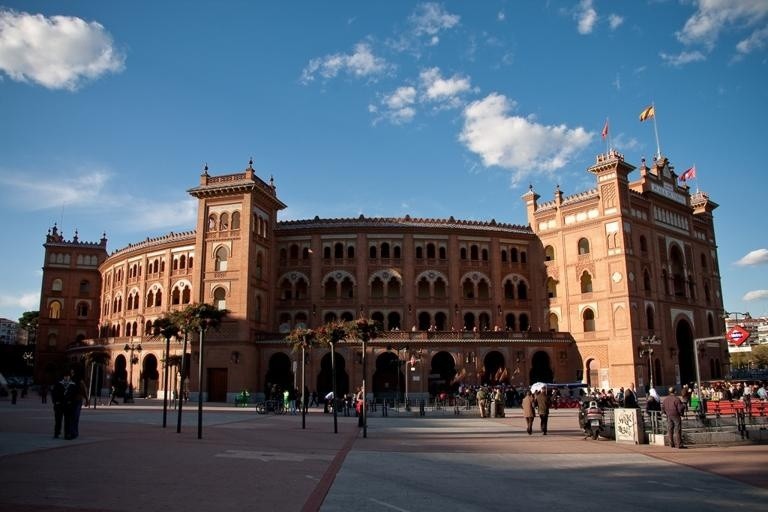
[312,304,316,317]
[466,352,470,363]
[455,304,459,316]
[408,304,412,315]
[360,304,364,315]
[472,352,476,363]
[498,304,503,315]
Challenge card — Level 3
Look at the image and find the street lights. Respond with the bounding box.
[637,336,662,388]
[123,343,143,403]
[718,310,752,380]
[21,352,33,394]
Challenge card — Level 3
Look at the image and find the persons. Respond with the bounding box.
[522,391,535,434]
[0,374,90,440]
[391,325,542,333]
[646,388,661,427]
[264,382,368,428]
[459,383,641,408]
[534,388,549,435]
[662,387,688,448]
[476,387,503,418]
[682,381,768,411]
[108,385,119,406]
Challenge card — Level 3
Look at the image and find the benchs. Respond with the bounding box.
[707,400,768,419]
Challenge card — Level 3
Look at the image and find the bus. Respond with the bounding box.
[700,379,762,388]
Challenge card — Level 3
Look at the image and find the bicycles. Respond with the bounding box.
[256,399,284,414]
[260,400,287,415]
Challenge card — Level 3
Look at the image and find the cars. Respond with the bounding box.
[0,372,34,397]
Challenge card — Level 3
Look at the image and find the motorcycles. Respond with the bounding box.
[578,398,605,440]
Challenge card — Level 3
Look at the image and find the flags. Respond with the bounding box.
[679,166,695,182]
[639,104,654,121]
[602,121,608,139]
